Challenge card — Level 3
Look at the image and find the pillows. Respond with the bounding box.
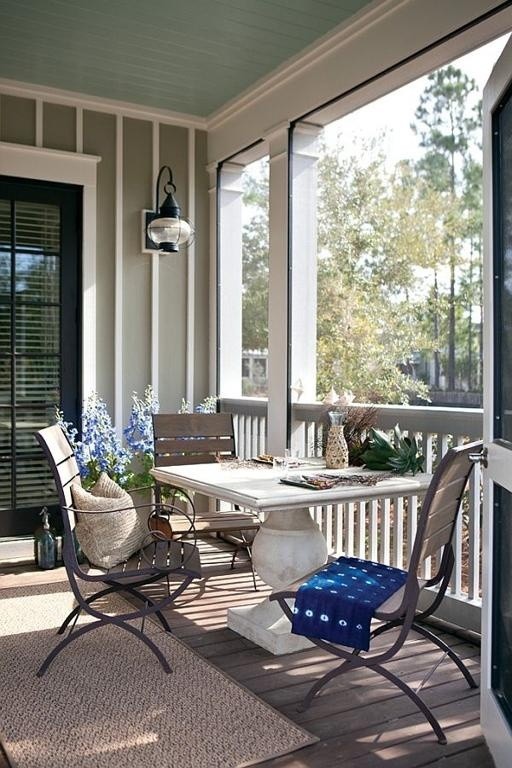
[71,472,154,570]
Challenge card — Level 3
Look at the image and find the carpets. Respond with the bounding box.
[0,580,320,768]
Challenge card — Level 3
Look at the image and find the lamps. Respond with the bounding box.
[141,165,198,254]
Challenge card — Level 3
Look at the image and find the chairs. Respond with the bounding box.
[34,425,202,677]
[151,413,262,598]
[269,441,484,744]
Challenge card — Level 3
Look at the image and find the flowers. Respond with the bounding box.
[56,385,216,501]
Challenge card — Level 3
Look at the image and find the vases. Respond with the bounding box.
[85,486,153,541]
[326,411,349,469]
